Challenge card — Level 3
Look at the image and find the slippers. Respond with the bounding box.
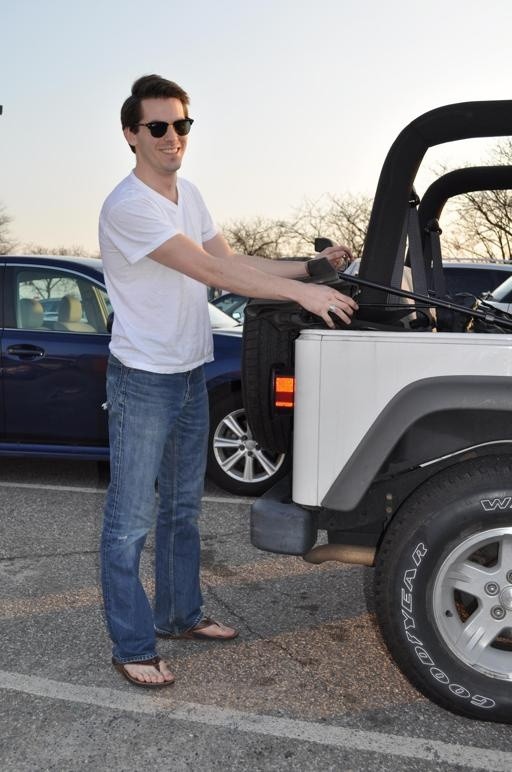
[111,654,175,687]
[158,616,239,640]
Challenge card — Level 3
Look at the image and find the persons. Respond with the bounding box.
[99,72,359,688]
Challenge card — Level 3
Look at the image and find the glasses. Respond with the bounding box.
[135,116,193,138]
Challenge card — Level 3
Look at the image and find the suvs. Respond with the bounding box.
[244,100,511,723]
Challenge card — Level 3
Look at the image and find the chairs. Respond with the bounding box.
[19,295,99,331]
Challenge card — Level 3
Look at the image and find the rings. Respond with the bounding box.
[328,303,336,312]
